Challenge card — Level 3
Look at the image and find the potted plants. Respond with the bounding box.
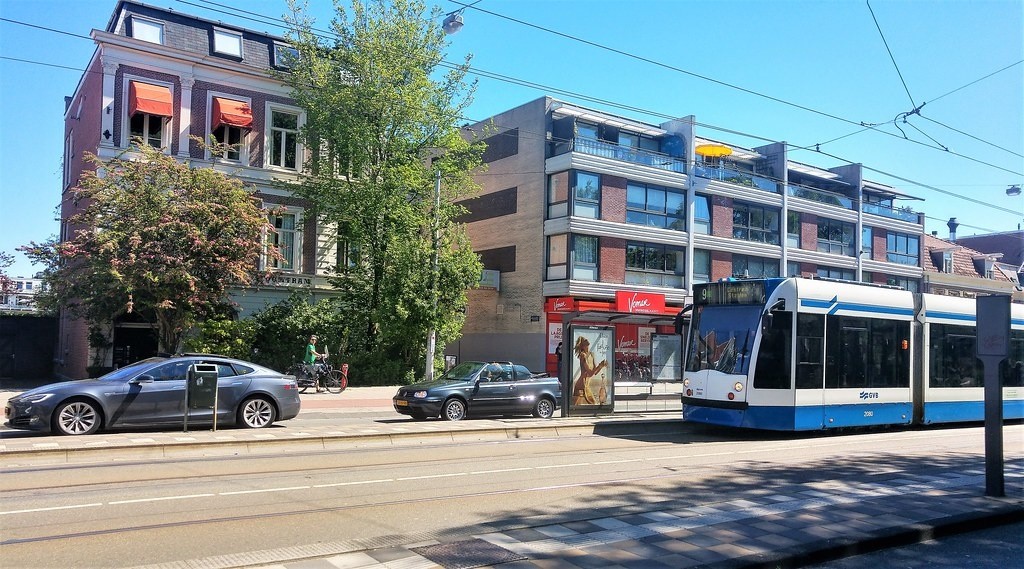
[86,327,113,378]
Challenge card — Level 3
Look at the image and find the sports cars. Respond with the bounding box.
[392,360,563,421]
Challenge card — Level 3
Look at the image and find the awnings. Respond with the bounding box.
[211,97,253,138]
[129,81,173,125]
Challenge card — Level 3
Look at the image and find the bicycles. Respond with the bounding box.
[284,354,348,394]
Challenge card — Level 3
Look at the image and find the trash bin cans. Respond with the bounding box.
[188,363,218,409]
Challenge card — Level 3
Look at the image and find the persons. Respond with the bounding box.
[305,335,327,393]
[573,336,608,404]
[554,341,562,382]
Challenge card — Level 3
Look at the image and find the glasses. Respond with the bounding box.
[311,339,318,342]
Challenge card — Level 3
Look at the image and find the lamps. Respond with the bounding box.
[442,9,464,35]
[1006,185,1021,196]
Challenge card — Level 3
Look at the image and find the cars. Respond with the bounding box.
[4,352,302,436]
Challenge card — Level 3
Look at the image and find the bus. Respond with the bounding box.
[674,274,1024,436]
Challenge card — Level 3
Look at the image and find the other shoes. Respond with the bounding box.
[316,390,324,393]
